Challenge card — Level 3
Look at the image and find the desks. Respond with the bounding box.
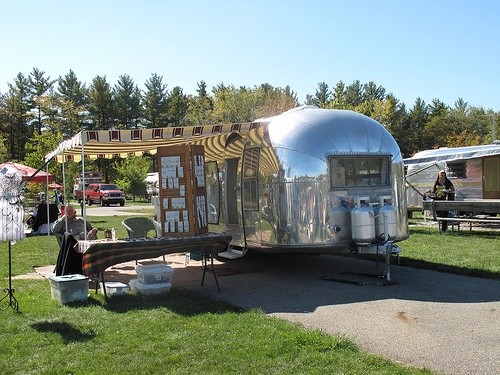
[74,232,233,304]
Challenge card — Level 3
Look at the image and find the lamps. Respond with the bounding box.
[225,132,245,147]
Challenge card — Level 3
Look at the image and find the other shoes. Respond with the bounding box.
[79,228,98,240]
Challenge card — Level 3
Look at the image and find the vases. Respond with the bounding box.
[447,192,455,200]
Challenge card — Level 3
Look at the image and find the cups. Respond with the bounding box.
[105,230,111,238]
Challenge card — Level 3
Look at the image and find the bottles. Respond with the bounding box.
[111,228,115,240]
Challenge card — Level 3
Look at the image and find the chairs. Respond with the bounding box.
[121,217,165,265]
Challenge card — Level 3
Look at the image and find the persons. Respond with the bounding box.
[52,190,64,205]
[433,172,455,233]
[301,188,324,245]
[26,197,49,232]
[51,206,97,241]
[262,194,298,244]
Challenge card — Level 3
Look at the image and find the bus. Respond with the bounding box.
[73,172,102,200]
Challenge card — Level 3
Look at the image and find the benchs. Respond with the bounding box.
[422,199,500,234]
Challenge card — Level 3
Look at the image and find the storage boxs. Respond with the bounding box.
[128,279,172,297]
[48,274,89,304]
[135,264,173,284]
[100,282,129,298]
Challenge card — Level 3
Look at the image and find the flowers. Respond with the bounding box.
[448,186,454,192]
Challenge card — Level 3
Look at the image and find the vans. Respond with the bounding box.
[143,171,159,200]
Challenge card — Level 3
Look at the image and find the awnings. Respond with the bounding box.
[403,145,500,164]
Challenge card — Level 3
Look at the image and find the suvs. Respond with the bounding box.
[85,183,126,207]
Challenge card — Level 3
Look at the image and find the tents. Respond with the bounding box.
[45,121,270,240]
[0,162,63,210]
